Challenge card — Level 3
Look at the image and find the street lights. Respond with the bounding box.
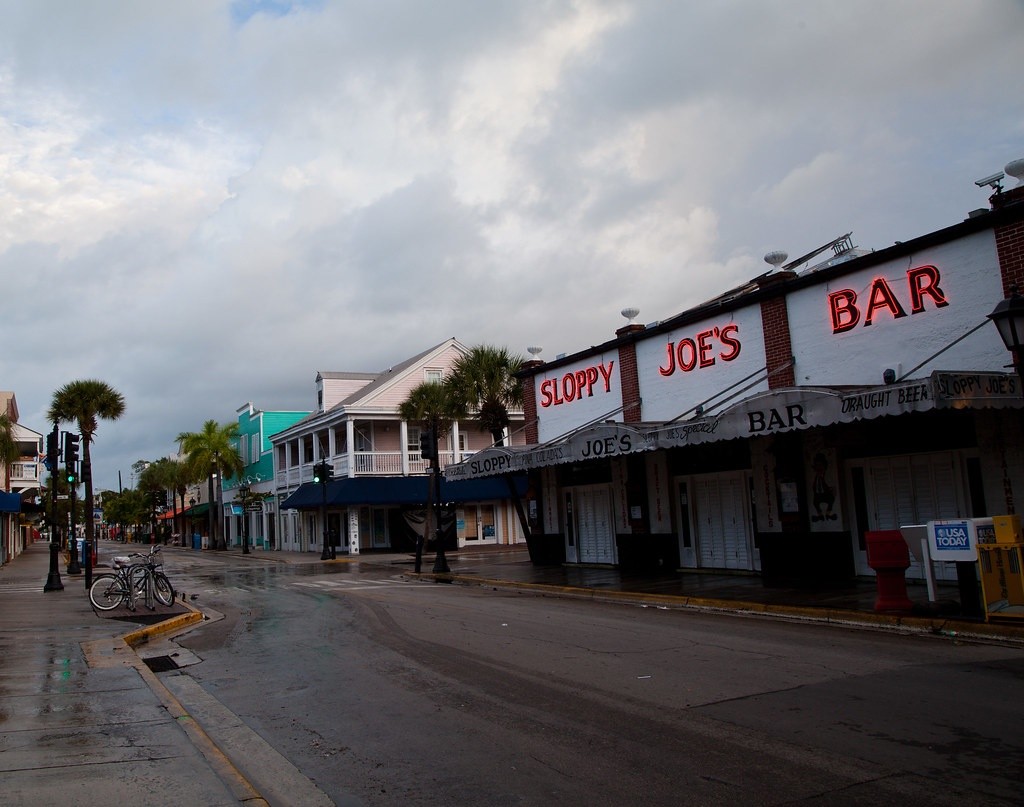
[188,497,196,550]
[239,483,252,554]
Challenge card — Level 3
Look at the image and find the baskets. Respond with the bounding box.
[142,553,164,566]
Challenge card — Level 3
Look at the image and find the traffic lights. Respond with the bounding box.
[65,432,80,483]
[313,463,323,484]
[326,464,335,485]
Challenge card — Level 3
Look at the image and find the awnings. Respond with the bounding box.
[156,506,191,520]
[177,501,218,518]
[445,368,1024,512]
[280,476,508,510]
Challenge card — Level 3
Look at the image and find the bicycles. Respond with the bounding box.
[88,544,175,610]
